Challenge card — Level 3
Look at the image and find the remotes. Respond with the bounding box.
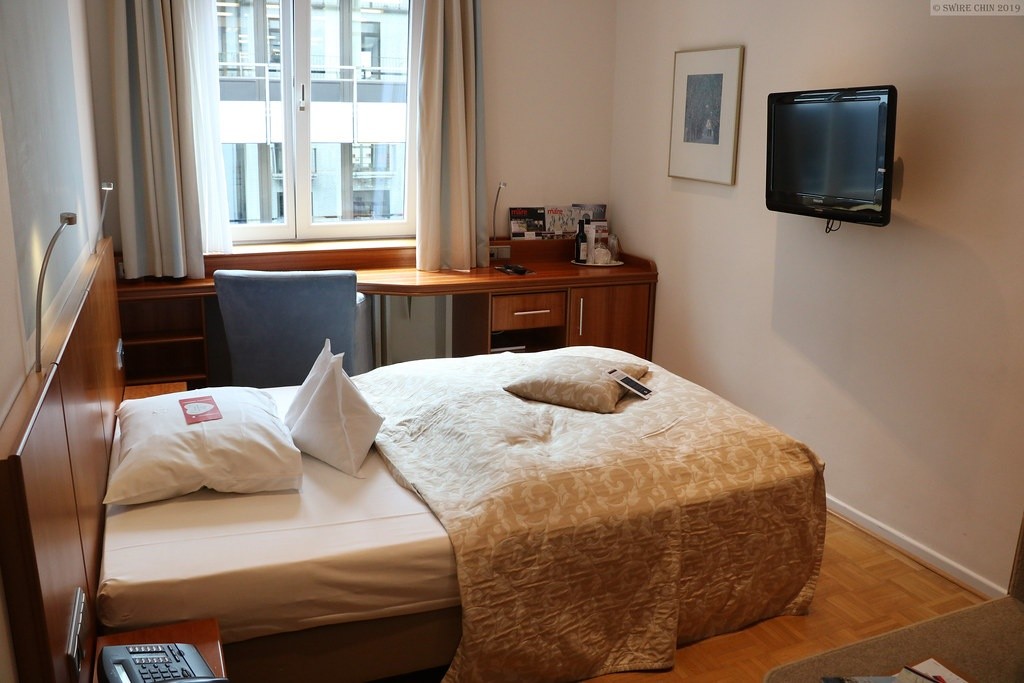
[504,264,527,274]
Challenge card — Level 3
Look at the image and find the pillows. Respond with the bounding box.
[290,351,387,479]
[102,386,302,506]
[502,355,649,414]
[283,338,381,431]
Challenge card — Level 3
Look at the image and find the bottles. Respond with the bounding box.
[577,218,595,264]
[575,219,588,264]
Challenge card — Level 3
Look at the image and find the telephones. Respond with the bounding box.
[97,643,229,683]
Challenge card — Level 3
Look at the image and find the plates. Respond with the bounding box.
[570,259,625,267]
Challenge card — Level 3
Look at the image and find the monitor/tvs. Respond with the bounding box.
[765,85,898,228]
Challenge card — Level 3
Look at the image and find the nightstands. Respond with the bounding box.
[92,617,228,683]
[122,381,188,402]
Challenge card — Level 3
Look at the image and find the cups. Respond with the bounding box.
[608,234,619,264]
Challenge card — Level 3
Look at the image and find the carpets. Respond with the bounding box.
[761,595,1024,683]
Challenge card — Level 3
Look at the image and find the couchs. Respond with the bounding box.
[213,269,375,390]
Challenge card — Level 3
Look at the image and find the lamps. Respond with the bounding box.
[34,212,78,373]
[489,181,507,257]
[93,181,114,254]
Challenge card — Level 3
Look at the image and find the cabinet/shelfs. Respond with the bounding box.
[115,275,208,392]
[451,282,657,363]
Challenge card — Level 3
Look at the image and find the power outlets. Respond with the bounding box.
[488,246,498,260]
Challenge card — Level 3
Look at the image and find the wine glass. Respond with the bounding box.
[593,224,610,264]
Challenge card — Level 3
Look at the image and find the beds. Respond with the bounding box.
[94,345,827,683]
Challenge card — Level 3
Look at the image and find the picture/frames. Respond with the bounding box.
[667,45,745,185]
